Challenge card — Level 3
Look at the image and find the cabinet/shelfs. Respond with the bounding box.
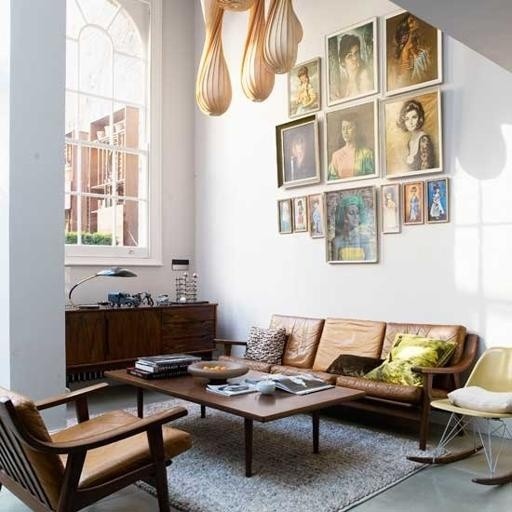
[64,303,218,387]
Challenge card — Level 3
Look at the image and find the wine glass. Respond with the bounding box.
[187,361,249,387]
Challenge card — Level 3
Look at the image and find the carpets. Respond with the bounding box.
[67,398,452,511]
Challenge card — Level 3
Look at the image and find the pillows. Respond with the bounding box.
[242,325,286,366]
[363,332,458,388]
[0,386,65,511]
[313,317,386,373]
[326,353,387,377]
[268,314,326,369]
[447,385,512,414]
[380,322,467,389]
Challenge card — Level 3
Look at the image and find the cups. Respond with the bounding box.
[257,381,275,396]
[156,295,169,306]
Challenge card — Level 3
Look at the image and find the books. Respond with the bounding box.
[126,353,202,380]
[243,374,334,395]
[206,384,259,397]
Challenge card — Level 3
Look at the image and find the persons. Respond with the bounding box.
[430,184,445,219]
[392,14,432,86]
[382,186,398,210]
[294,66,317,112]
[395,98,436,170]
[280,200,293,231]
[330,196,376,261]
[407,186,421,223]
[334,34,374,97]
[330,113,375,178]
[291,134,314,179]
[309,198,323,234]
[295,199,306,230]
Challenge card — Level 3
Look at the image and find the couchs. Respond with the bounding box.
[213,333,480,451]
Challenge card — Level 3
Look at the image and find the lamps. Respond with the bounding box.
[194,0,303,116]
[68,267,138,308]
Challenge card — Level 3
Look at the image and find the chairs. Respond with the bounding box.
[405,346,512,485]
[0,381,193,512]
